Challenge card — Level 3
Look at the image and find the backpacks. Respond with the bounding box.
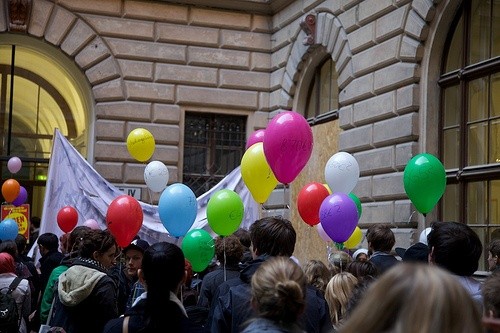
[0,277,25,333]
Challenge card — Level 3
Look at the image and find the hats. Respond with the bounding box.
[122,240,149,254]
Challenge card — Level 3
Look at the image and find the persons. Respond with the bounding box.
[0,215,500,333]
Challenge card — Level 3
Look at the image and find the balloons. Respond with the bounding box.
[106,194,144,248]
[144,161,169,193]
[246,128,264,149]
[0,219,18,241]
[263,111,314,185]
[83,219,99,229]
[126,128,155,162]
[57,206,78,234]
[180,228,216,273]
[7,157,22,174]
[206,189,244,237]
[402,152,447,215]
[2,179,28,207]
[297,152,363,250]
[158,183,198,238]
[240,142,280,205]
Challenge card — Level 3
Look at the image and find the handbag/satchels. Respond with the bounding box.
[39,324,51,333]
